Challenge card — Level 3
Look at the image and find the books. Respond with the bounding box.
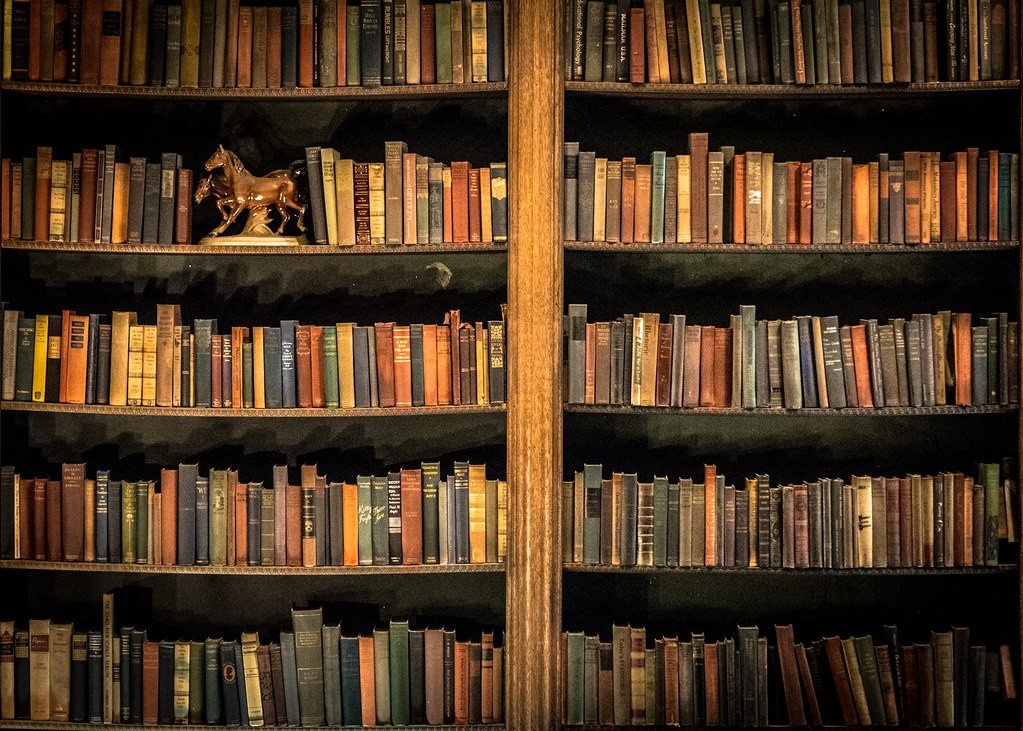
[0,295,1021,410]
[0,129,1023,248]
[2,459,1023,568]
[0,0,1023,90]
[0,590,1023,730]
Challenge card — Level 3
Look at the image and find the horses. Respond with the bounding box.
[203,143,310,237]
[192,167,307,238]
